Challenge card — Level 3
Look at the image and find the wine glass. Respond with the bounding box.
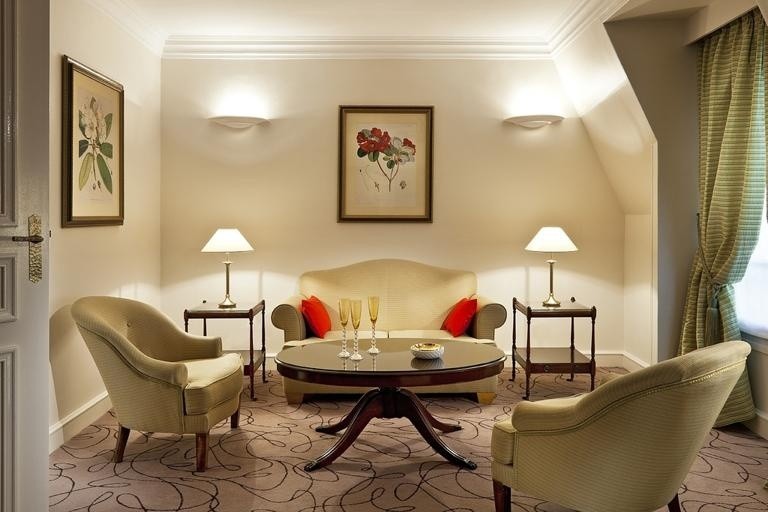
[368,295,381,354]
[336,297,352,359]
[349,299,364,362]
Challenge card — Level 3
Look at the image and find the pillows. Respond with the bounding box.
[441,298,477,337]
[301,296,331,338]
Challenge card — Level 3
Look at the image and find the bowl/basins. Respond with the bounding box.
[410,343,445,359]
[411,356,444,371]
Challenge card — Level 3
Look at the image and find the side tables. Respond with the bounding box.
[513,297,596,399]
[184,299,266,398]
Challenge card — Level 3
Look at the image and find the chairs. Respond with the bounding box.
[71,295,245,472]
[492,340,751,512]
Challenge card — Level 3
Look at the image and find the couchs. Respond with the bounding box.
[271,258,507,405]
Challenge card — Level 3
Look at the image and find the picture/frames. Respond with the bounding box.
[62,55,124,228]
[338,105,434,223]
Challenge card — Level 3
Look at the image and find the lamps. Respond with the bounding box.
[210,116,268,129]
[201,227,254,307]
[504,114,564,129]
[526,226,578,307]
